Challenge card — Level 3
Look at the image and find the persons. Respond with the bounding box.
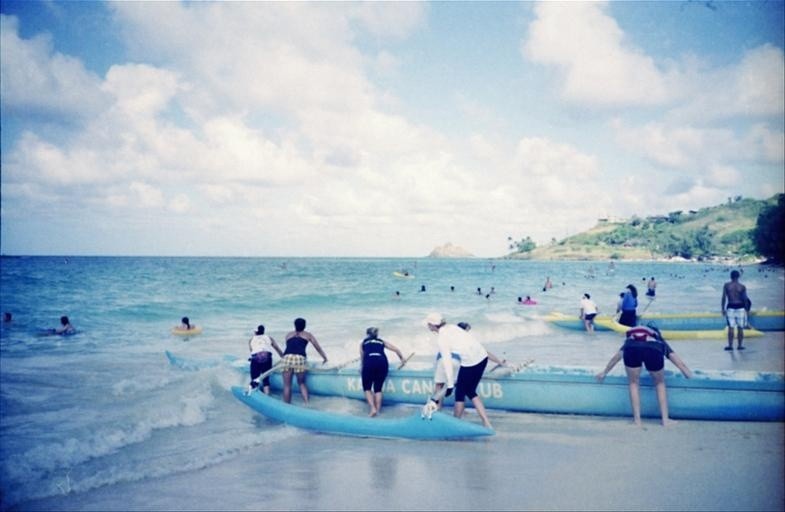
[3,312,11,321]
[55,315,75,334]
[434,322,504,410]
[181,317,192,331]
[248,325,284,396]
[360,326,407,418]
[279,317,328,404]
[421,312,493,428]
[596,320,694,428]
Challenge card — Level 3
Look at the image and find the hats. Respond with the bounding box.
[421,311,444,326]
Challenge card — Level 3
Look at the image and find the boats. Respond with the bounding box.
[219,355,785,440]
[164,349,241,370]
[541,309,785,342]
